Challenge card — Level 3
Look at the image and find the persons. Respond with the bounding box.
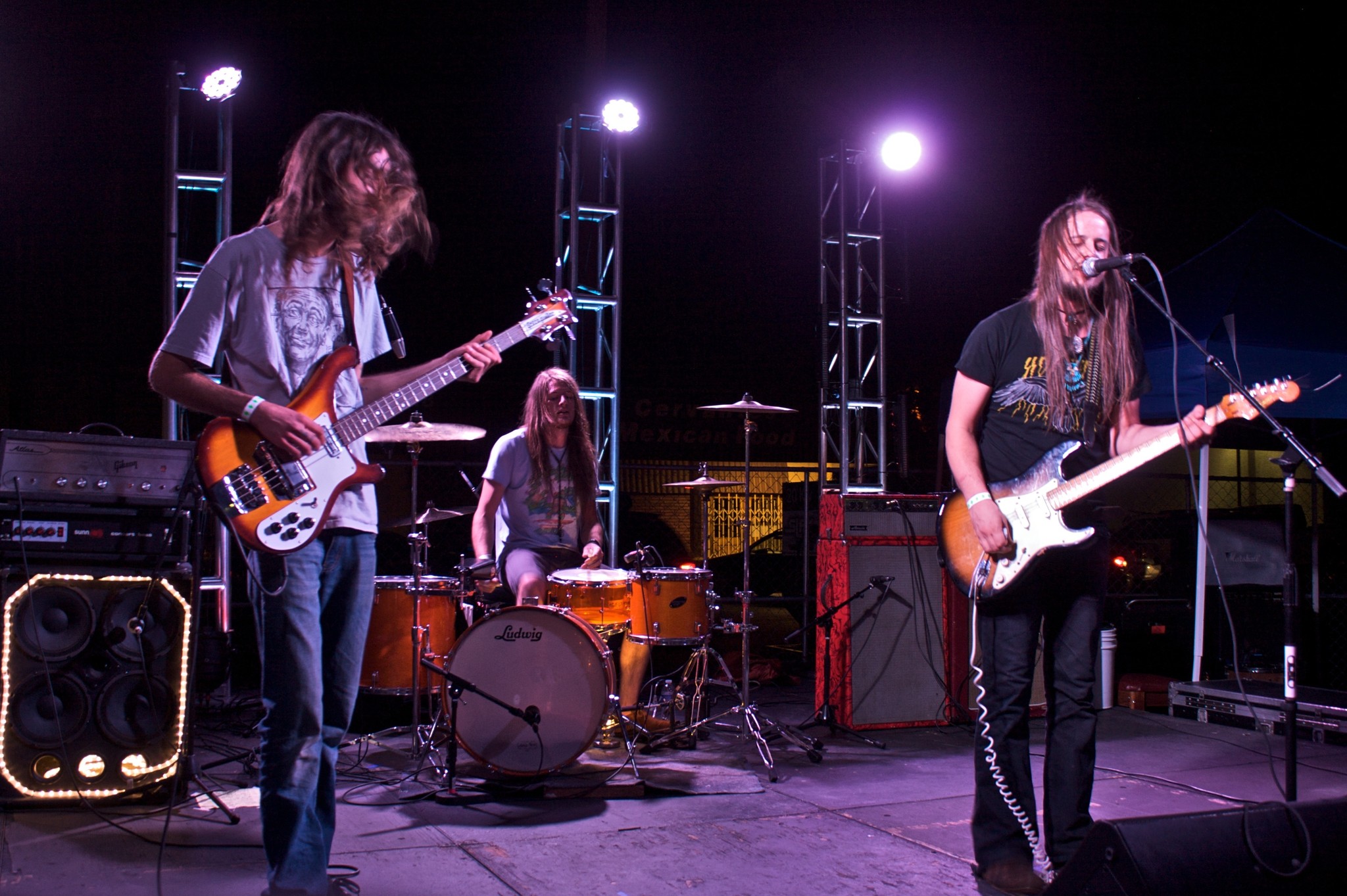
[472,368,681,738]
[945,189,1216,896]
[149,113,502,896]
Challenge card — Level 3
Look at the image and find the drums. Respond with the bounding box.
[548,566,632,634]
[440,604,617,776]
[627,566,713,645]
[359,575,459,696]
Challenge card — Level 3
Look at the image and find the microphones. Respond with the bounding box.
[526,705,541,734]
[1081,252,1146,278]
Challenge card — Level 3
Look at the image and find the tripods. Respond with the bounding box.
[338,445,459,790]
[641,411,825,782]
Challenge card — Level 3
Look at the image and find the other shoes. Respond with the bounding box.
[326,875,361,896]
[970,854,1049,896]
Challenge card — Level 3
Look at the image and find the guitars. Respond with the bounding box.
[936,373,1303,604]
[196,278,580,554]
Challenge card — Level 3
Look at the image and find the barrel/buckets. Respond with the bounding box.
[1100,627,1118,709]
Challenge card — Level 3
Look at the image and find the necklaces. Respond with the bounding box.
[546,444,568,544]
[1056,305,1095,392]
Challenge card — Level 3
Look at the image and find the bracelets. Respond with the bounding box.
[965,492,993,510]
[237,393,264,427]
[587,539,601,548]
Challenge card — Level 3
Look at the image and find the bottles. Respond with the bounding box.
[661,680,675,720]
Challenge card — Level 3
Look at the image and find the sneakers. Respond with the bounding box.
[613,702,680,738]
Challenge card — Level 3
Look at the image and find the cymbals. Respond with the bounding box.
[696,400,799,414]
[364,422,486,443]
[384,505,480,528]
[662,476,746,491]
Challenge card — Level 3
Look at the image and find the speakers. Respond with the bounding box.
[815,540,1047,732]
[0,562,196,811]
[1037,794,1347,896]
